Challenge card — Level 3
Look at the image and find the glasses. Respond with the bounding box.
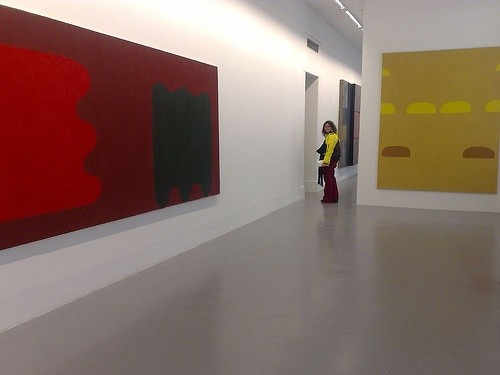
[325,126,331,127]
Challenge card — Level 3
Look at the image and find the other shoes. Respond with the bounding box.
[321,199,338,203]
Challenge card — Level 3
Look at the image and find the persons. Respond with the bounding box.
[316,120,341,203]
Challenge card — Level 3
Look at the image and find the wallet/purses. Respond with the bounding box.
[317,160,329,166]
